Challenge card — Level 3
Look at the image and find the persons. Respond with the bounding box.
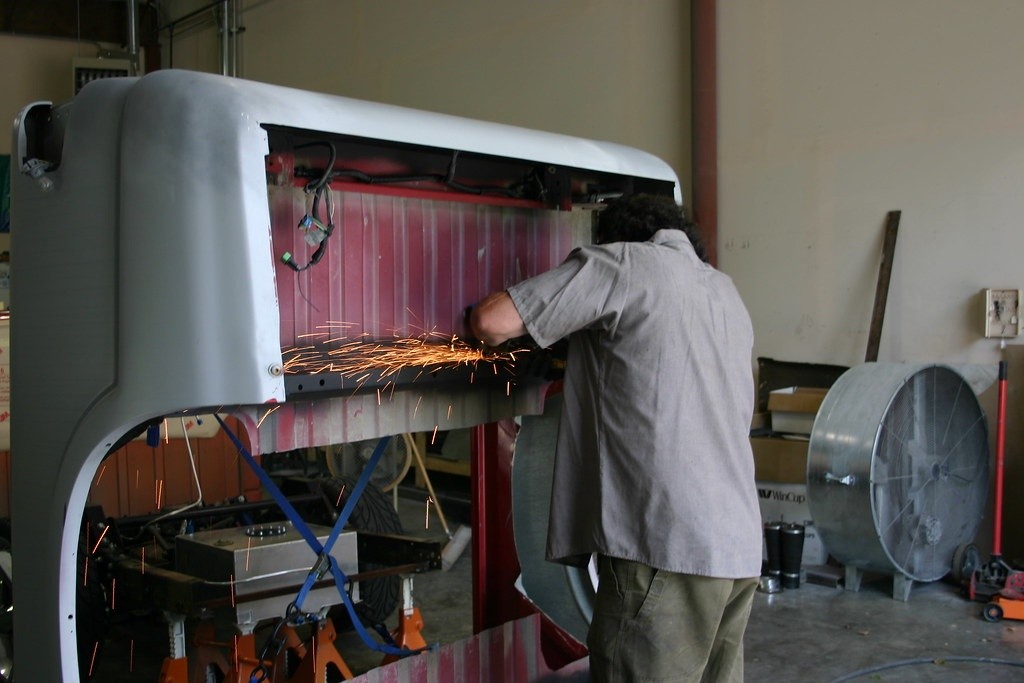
[471,193,763,681]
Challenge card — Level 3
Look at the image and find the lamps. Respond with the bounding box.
[19,157,54,194]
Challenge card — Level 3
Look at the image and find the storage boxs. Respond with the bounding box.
[746,356,853,568]
